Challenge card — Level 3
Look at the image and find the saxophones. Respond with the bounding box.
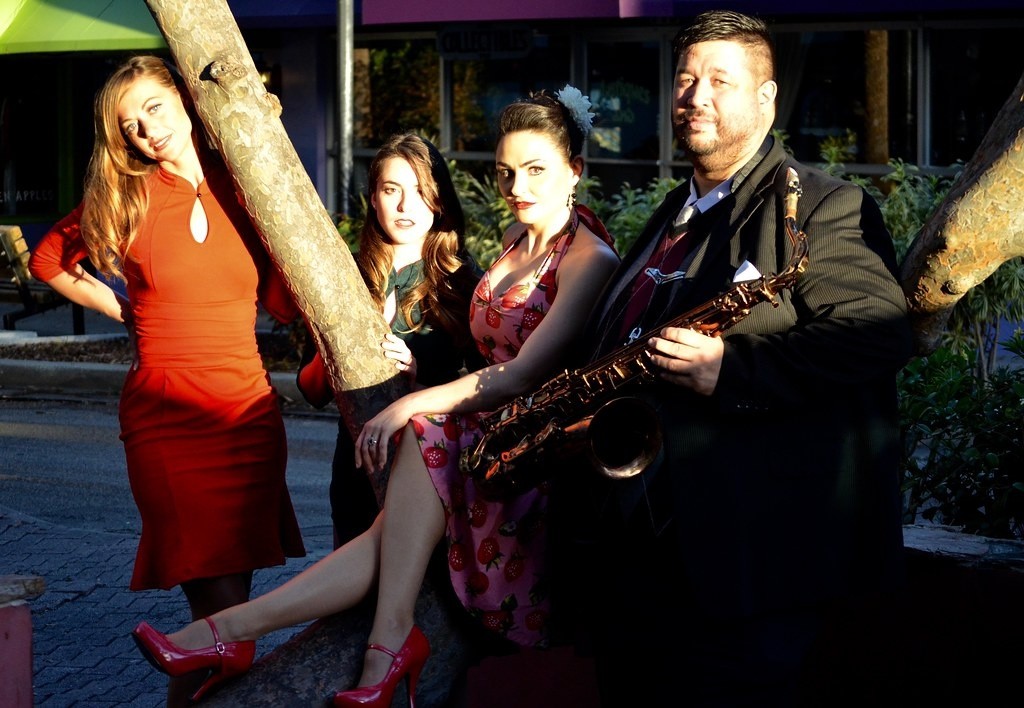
[447,164,814,521]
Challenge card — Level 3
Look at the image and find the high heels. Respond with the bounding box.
[335,624,431,708]
[132,616,255,701]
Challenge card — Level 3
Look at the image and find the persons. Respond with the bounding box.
[135,83,620,708]
[30,56,311,708]
[298,130,487,555]
[539,10,919,708]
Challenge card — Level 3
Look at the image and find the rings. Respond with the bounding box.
[369,438,378,446]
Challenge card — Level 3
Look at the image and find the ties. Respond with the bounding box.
[674,205,698,226]
